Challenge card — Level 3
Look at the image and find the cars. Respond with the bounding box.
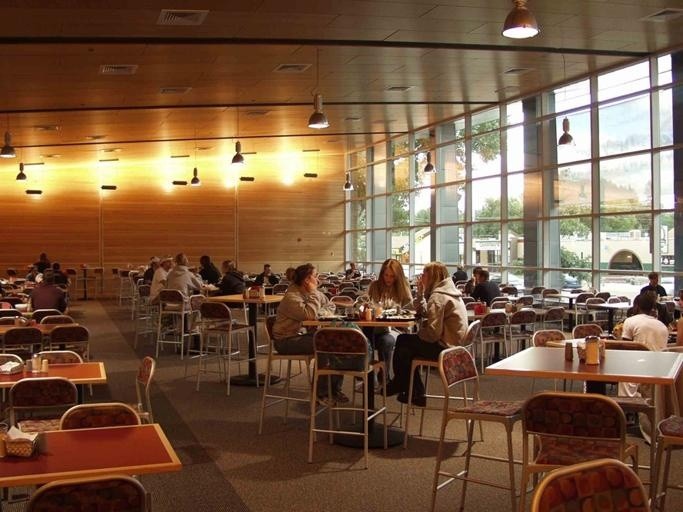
[489,273,522,287]
[560,275,581,289]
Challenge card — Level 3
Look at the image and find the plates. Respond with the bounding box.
[385,310,418,321]
[343,318,359,321]
[322,316,345,320]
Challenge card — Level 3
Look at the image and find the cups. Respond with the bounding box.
[0,422,10,459]
[338,306,356,319]
[201,279,209,288]
[29,318,36,327]
[243,289,249,299]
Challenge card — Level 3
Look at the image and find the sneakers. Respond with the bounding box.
[315,391,350,409]
[355,378,390,394]
[379,379,427,407]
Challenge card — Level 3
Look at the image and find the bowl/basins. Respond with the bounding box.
[14,304,27,308]
[79,263,87,268]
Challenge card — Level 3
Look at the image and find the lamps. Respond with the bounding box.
[189,108,203,188]
[302,43,331,130]
[555,51,580,147]
[422,105,438,174]
[15,113,29,183]
[501,0,542,41]
[342,134,356,191]
[231,107,246,164]
[0,112,16,159]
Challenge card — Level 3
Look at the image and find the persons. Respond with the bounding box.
[279,267,297,285]
[255,264,280,314]
[144,252,244,355]
[452,266,468,286]
[380,263,468,407]
[352,259,414,392]
[469,271,501,307]
[465,267,483,296]
[273,262,349,407]
[26,253,67,323]
[611,271,683,424]
[346,262,361,280]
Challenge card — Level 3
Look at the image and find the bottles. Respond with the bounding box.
[658,293,678,303]
[14,315,20,326]
[397,306,401,314]
[359,297,383,321]
[25,353,48,373]
[576,335,601,365]
[505,300,518,313]
[565,340,573,361]
[249,285,267,299]
[474,296,491,314]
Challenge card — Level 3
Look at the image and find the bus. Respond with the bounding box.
[660,254,674,265]
